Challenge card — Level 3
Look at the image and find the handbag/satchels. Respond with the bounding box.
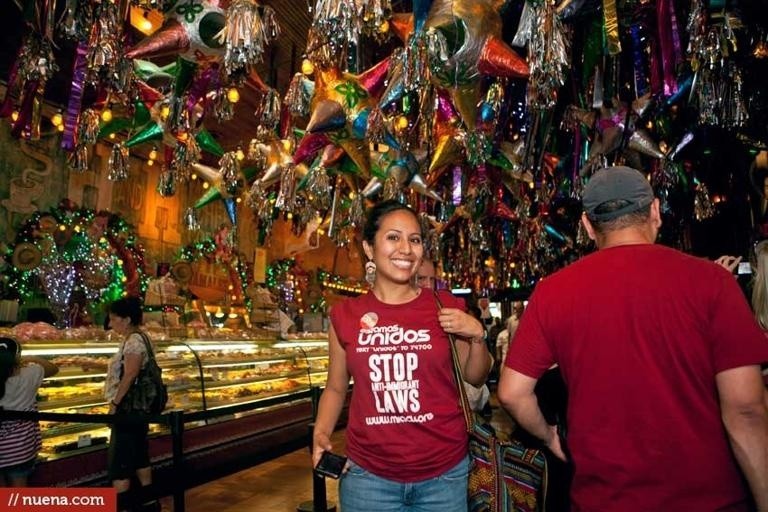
[118,356,168,414]
[466,421,549,510]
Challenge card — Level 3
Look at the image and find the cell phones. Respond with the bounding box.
[729,260,753,276]
[315,449,347,479]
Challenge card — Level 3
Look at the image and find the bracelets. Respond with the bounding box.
[111,400,118,407]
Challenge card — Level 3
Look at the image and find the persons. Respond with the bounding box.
[311,199,490,512]
[104,296,161,511]
[713,239,768,330]
[495,163,767,512]
[0,337,59,487]
[416,257,528,413]
[510,365,575,512]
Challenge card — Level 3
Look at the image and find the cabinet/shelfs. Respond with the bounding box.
[19,331,354,499]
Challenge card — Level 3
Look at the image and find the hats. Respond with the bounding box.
[582,165,652,220]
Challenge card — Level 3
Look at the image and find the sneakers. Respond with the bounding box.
[128,501,162,511]
